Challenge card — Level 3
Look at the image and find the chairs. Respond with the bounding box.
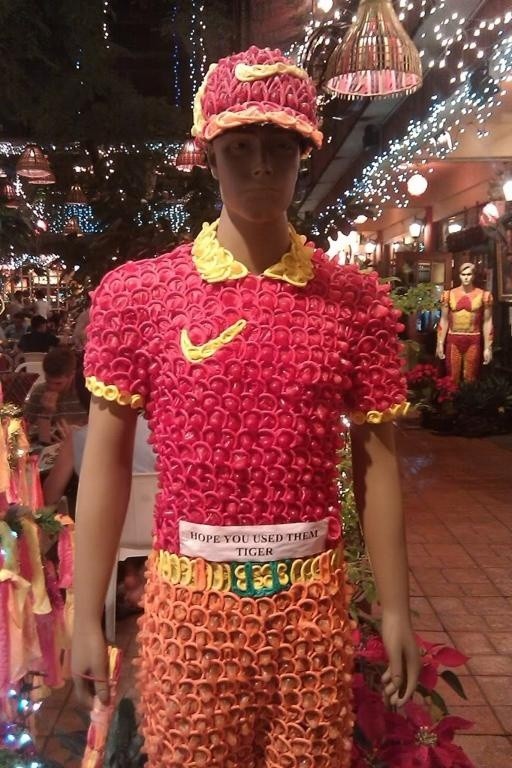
[97,474,163,642]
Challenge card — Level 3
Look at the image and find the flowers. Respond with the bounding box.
[404,363,460,409]
[350,618,476,768]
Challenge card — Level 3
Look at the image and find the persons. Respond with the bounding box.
[0,290,157,506]
[436,262,493,387]
[71,47,420,768]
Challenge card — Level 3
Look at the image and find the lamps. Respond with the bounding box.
[409,215,427,239]
[0,142,57,210]
[175,139,210,171]
[320,2,425,100]
